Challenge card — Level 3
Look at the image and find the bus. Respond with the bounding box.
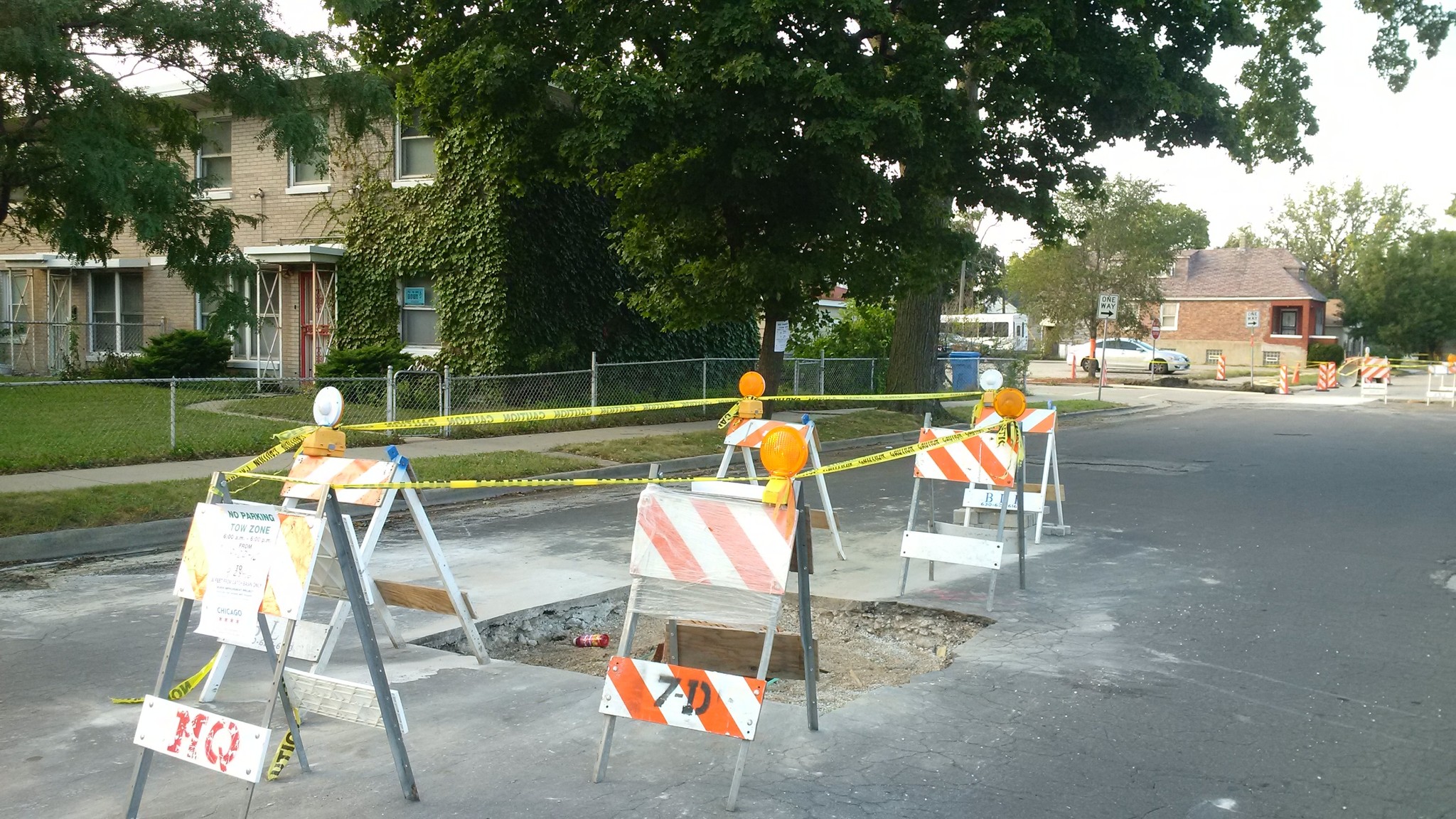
[935,313,1029,352]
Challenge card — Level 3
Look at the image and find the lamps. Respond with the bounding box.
[250,188,264,201]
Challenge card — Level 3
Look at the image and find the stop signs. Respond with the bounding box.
[1151,318,1162,338]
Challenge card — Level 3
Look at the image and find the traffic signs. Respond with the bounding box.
[1096,295,1119,319]
[1245,310,1259,328]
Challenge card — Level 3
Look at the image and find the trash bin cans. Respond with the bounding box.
[936,345,951,390]
[948,351,982,393]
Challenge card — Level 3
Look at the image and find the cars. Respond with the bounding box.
[1068,338,1190,376]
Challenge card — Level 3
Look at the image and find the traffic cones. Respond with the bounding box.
[1289,364,1301,384]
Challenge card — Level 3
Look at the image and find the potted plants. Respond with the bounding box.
[0,324,25,375]
